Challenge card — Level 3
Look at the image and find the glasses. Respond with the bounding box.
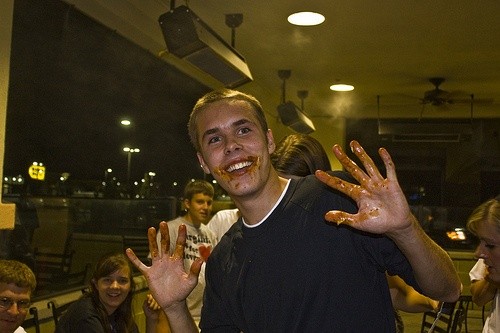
[0,296,31,313]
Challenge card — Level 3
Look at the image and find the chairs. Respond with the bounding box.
[420,294,464,333]
[122,226,152,271]
[32,232,74,286]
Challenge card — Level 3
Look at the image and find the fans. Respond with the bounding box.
[395,76,494,108]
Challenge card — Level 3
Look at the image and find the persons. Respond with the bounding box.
[147,131,439,333]
[53,251,162,333]
[126,88,462,333]
[0,259,36,333]
[466,195,500,333]
